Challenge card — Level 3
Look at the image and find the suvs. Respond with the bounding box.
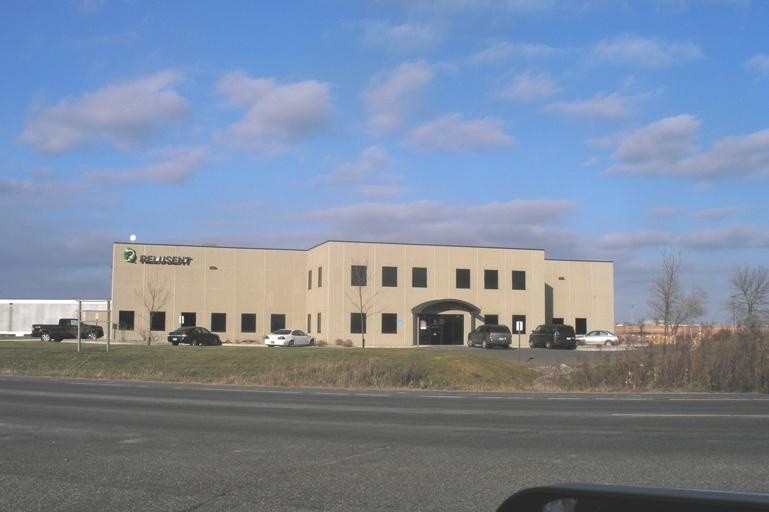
[467,323,514,348]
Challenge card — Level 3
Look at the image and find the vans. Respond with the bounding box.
[526,321,576,350]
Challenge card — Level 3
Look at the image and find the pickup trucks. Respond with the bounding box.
[31,318,103,343]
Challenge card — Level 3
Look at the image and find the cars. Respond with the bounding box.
[574,327,626,348]
[264,327,318,347]
[167,324,223,348]
